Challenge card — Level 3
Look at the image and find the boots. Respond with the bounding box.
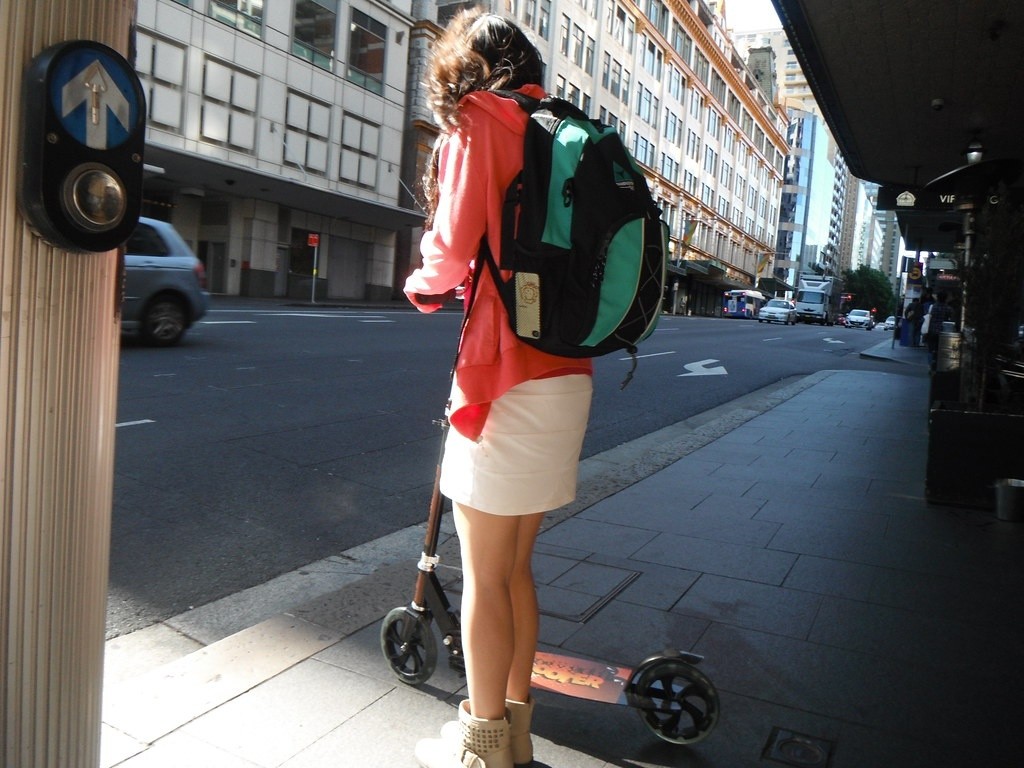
[442,695,534,766]
[416,704,514,768]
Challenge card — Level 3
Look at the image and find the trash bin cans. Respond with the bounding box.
[900,317,925,347]
[700,306,706,316]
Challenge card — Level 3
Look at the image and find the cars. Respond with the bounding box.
[833,314,845,325]
[758,299,797,325]
[883,315,896,331]
[845,309,874,331]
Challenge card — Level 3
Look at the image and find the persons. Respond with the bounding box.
[402,6,594,768]
[905,286,958,352]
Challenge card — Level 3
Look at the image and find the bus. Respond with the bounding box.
[723,290,767,320]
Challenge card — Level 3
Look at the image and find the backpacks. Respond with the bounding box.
[477,89,668,359]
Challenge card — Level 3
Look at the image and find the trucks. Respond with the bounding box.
[792,275,844,326]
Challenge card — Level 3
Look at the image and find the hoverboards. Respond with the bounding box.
[379,293,723,747]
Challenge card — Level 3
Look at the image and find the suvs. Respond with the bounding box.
[121,215,209,347]
